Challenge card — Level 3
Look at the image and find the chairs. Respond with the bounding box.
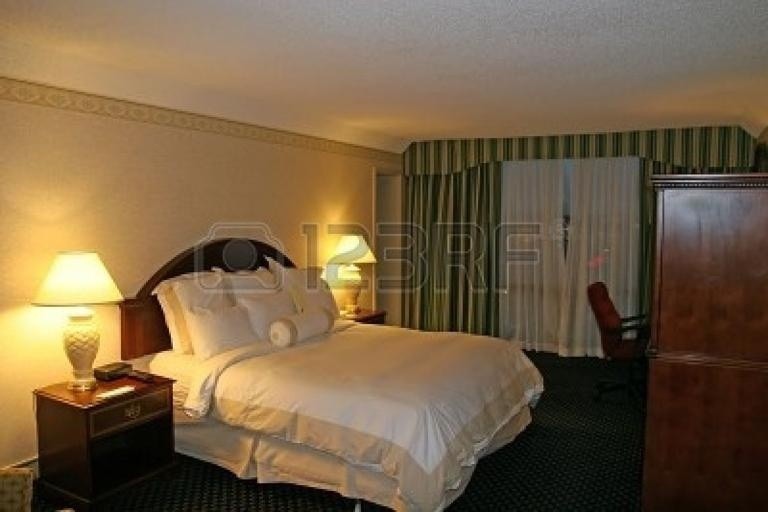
[0,468,33,511]
[588,281,647,409]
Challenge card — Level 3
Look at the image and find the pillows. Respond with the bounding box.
[149,267,336,360]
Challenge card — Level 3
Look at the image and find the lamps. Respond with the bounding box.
[329,234,378,315]
[33,251,126,392]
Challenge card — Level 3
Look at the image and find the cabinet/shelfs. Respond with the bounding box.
[643,173,767,512]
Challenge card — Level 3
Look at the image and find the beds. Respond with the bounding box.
[116,238,545,511]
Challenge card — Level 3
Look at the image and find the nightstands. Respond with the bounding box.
[346,307,386,324]
[36,370,176,508]
